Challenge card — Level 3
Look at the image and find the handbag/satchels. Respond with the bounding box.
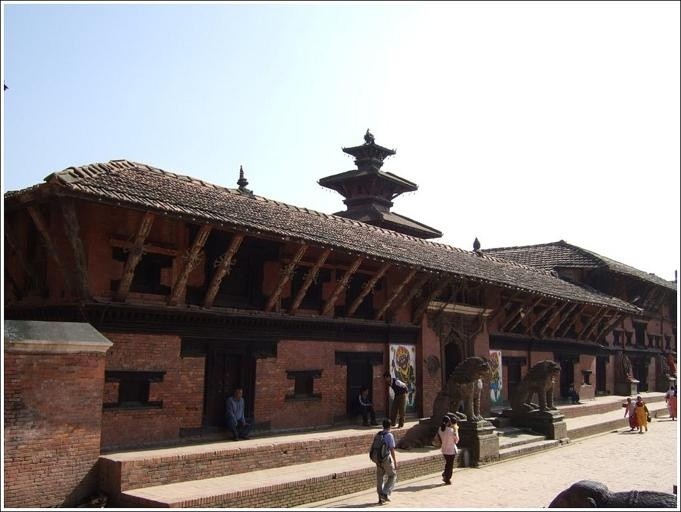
[432,433,442,448]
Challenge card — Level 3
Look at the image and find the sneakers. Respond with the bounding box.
[442,473,451,485]
[631,427,648,433]
[378,494,391,505]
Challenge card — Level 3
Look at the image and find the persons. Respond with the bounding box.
[382,371,409,428]
[450,417,459,432]
[223,387,253,441]
[351,387,378,427]
[437,415,458,485]
[566,384,581,404]
[375,417,398,505]
[624,397,637,431]
[664,384,677,420]
[634,396,649,433]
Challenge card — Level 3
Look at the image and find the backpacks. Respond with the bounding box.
[369,431,391,464]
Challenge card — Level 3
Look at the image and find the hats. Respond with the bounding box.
[383,419,391,427]
